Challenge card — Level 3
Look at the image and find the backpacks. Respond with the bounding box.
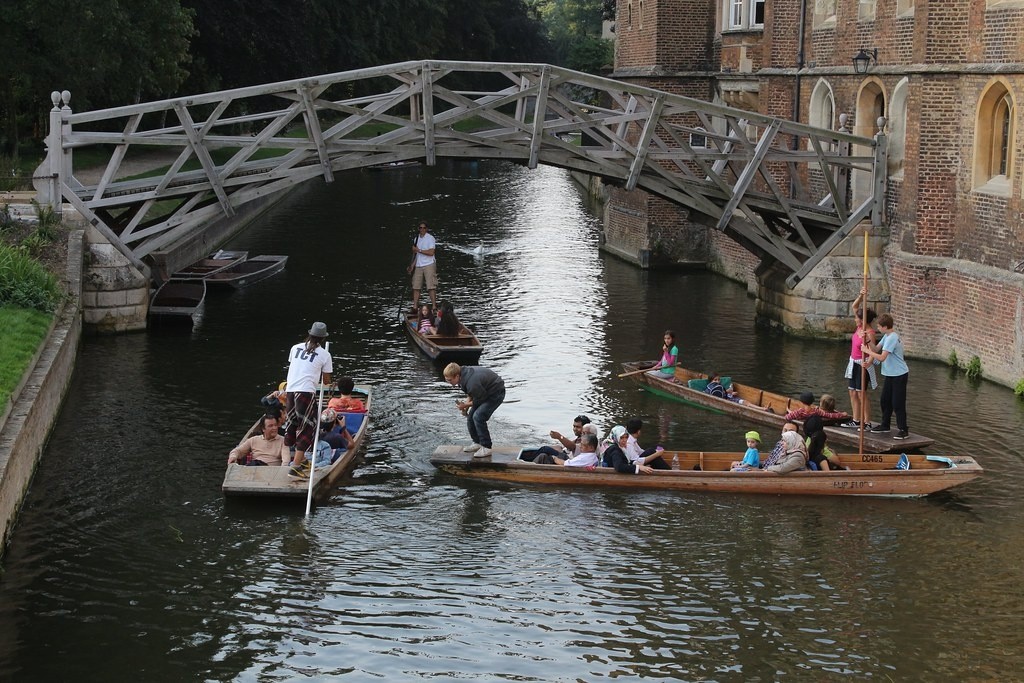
[704,382,725,398]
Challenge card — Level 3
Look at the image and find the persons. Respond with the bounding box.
[730,415,846,472]
[785,391,847,421]
[417,299,459,336]
[407,221,438,316]
[222,377,363,473]
[532,416,700,475]
[841,289,877,431]
[703,372,771,412]
[861,314,909,439]
[647,330,678,383]
[443,363,505,457]
[284,322,333,480]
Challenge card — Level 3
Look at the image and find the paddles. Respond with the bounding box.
[303,341,331,520]
[617,361,682,378]
[397,230,423,320]
[454,397,469,417]
[858,231,869,454]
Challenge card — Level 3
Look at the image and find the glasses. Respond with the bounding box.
[419,226,427,229]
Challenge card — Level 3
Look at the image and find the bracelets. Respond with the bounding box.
[561,435,564,439]
[339,426,346,434]
[866,340,871,343]
[419,250,422,253]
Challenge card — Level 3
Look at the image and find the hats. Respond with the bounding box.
[320,407,338,423]
[307,322,330,337]
[799,390,816,403]
[745,430,763,444]
[802,414,824,437]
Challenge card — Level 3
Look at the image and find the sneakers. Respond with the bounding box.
[893,427,910,440]
[857,422,872,432]
[870,424,891,433]
[473,446,493,458]
[287,466,310,481]
[298,459,320,472]
[463,442,481,452]
[840,420,861,428]
[896,453,909,470]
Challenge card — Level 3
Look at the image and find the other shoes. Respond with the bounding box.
[407,307,420,314]
[432,309,438,317]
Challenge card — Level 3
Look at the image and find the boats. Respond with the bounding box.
[171,250,248,278]
[403,309,483,364]
[361,160,421,174]
[621,360,936,454]
[429,445,985,497]
[222,382,372,505]
[148,278,207,327]
[202,254,289,290]
